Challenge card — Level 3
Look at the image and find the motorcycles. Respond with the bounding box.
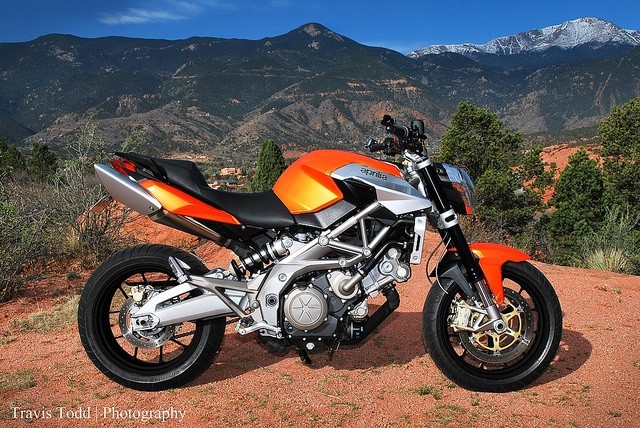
[77,114,562,392]
